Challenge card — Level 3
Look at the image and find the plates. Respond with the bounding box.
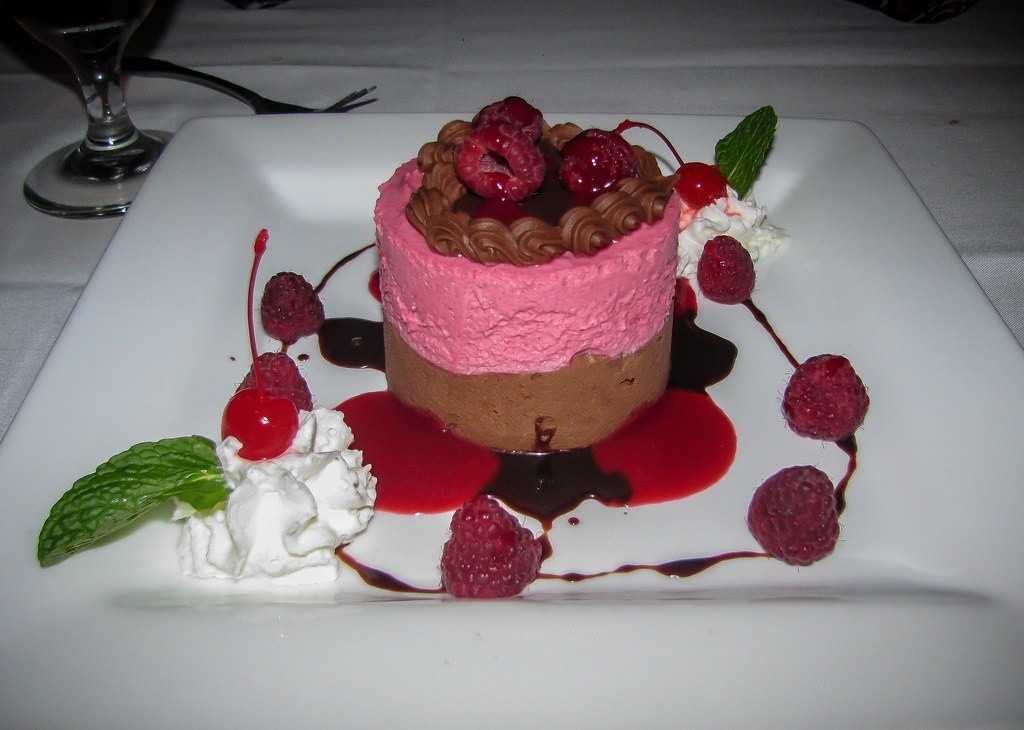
[0,116,1024,730]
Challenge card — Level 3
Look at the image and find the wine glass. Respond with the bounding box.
[8,0,174,221]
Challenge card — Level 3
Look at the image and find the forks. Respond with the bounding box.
[121,55,377,114]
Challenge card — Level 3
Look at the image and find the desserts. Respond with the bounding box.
[671,161,789,268]
[176,354,377,579]
[375,92,681,447]
